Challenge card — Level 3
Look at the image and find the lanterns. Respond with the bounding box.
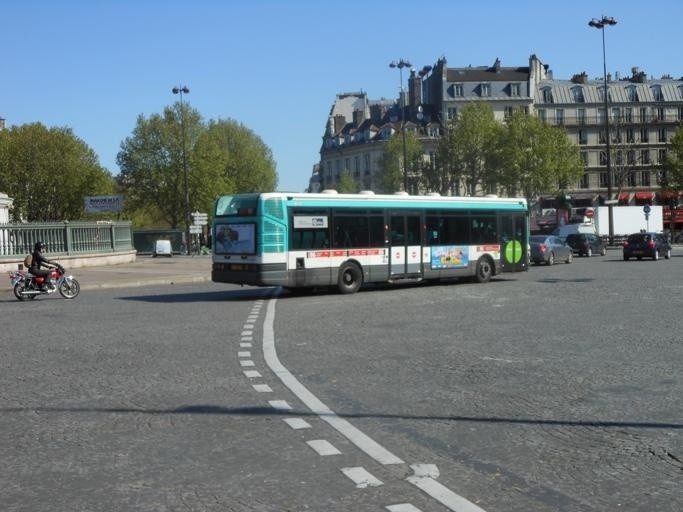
[584,209,594,219]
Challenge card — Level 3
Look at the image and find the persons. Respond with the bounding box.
[225,227,239,245]
[214,226,234,254]
[28,241,58,290]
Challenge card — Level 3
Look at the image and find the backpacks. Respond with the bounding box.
[24,255,33,267]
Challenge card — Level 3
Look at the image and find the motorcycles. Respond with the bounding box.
[6,257,82,302]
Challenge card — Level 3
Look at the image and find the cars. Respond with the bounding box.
[623,232,672,261]
[529,235,574,265]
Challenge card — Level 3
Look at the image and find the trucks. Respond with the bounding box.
[559,203,665,245]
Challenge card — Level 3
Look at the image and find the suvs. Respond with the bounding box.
[566,233,607,258]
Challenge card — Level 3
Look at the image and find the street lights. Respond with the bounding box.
[588,12,618,247]
[389,57,413,191]
[170,82,192,253]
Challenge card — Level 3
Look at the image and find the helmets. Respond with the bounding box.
[36,243,45,251]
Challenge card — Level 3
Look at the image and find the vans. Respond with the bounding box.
[151,239,173,258]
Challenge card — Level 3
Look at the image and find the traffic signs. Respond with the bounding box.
[190,229,202,233]
[190,213,208,216]
[194,220,208,225]
[194,216,208,220]
[189,225,202,229]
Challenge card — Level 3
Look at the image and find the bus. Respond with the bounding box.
[212,190,531,294]
